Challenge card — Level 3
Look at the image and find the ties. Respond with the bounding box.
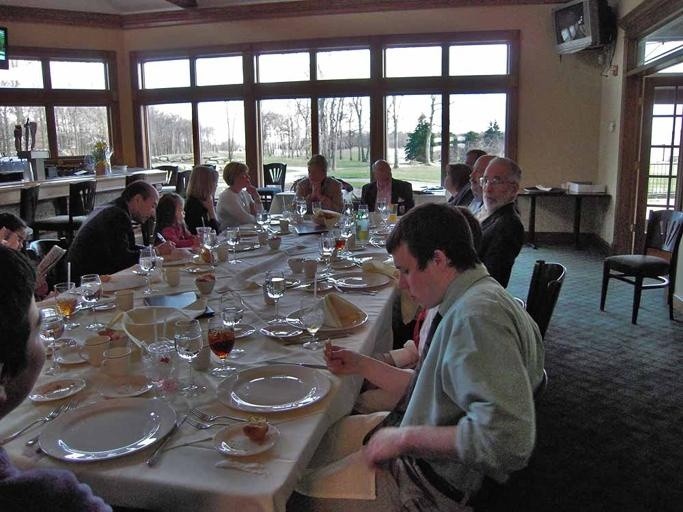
[363,313,442,443]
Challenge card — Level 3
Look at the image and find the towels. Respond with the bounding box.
[109,293,207,338]
[302,291,365,332]
[358,258,425,327]
[287,401,396,503]
[346,384,404,421]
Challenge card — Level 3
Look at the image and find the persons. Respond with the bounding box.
[63,180,159,290]
[0,212,49,298]
[183,165,220,237]
[216,162,264,232]
[154,193,201,249]
[1,242,114,512]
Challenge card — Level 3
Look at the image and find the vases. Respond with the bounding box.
[94,164,110,176]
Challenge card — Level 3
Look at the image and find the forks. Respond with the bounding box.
[185,416,231,429]
[1,398,79,444]
[190,407,270,424]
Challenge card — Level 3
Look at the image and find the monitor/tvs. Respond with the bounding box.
[0,27,9,69]
[555,0,597,54]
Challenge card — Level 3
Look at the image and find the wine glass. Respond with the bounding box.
[300,295,325,349]
[218,291,244,358]
[38,304,64,375]
[139,195,399,323]
[207,315,238,378]
[173,320,203,392]
[52,282,78,331]
[78,274,104,332]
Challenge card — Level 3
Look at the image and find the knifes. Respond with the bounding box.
[265,360,328,369]
[282,332,351,345]
[145,414,188,465]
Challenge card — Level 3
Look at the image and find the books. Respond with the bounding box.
[37,244,67,278]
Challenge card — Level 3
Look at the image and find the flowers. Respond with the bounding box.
[89,137,113,168]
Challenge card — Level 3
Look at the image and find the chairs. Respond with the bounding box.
[591,208,683,326]
[511,254,568,345]
[465,354,552,511]
[253,162,287,212]
[11,163,220,299]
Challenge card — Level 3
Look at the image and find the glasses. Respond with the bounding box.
[479,177,509,186]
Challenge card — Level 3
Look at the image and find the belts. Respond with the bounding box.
[417,459,471,504]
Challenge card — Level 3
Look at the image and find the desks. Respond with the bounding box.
[513,184,612,251]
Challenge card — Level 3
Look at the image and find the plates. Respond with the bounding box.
[37,398,177,464]
[285,307,368,331]
[215,361,331,412]
[44,337,87,365]
[93,304,116,311]
[29,377,84,402]
[348,249,397,269]
[327,268,398,293]
[260,324,302,339]
[96,374,152,398]
[214,420,279,457]
[216,242,261,251]
[232,324,255,339]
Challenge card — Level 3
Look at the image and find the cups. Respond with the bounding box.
[190,345,212,371]
[268,219,288,253]
[114,291,134,313]
[22,162,33,183]
[78,329,132,377]
[139,338,175,402]
[288,256,322,277]
